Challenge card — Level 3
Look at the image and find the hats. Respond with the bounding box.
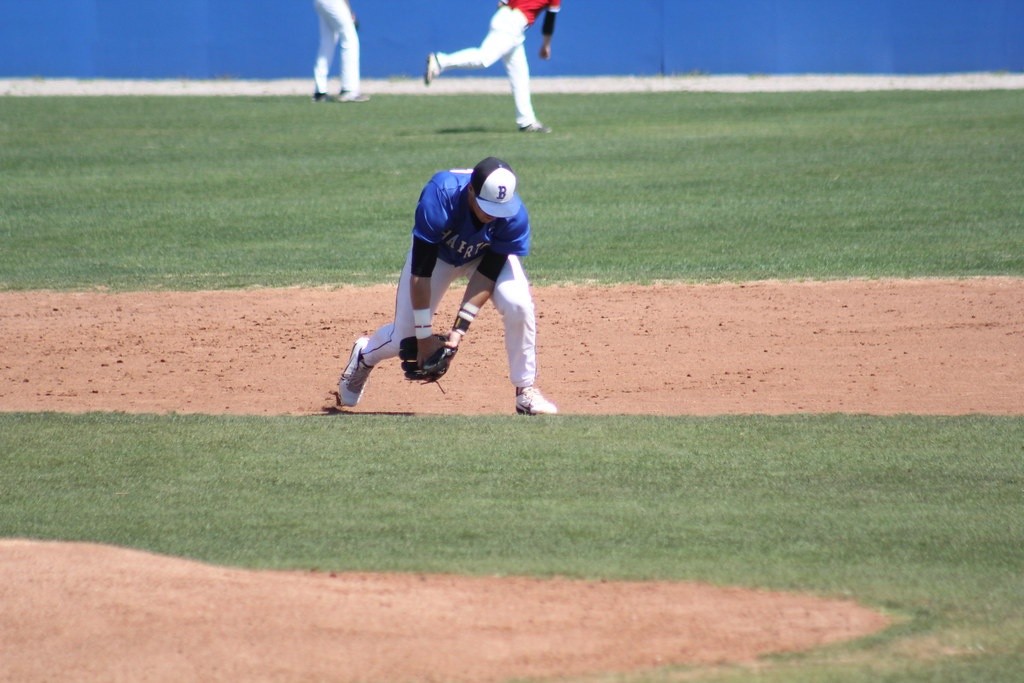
[470,157,522,218]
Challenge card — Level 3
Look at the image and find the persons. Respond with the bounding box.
[325,156,559,415]
[426,0,563,134]
[313,1,370,104]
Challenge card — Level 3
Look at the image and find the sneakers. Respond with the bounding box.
[425,52,440,85]
[336,90,371,103]
[515,387,558,415]
[339,337,375,407]
[310,93,337,103]
[519,123,552,134]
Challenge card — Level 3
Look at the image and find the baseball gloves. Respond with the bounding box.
[398,333,459,395]
